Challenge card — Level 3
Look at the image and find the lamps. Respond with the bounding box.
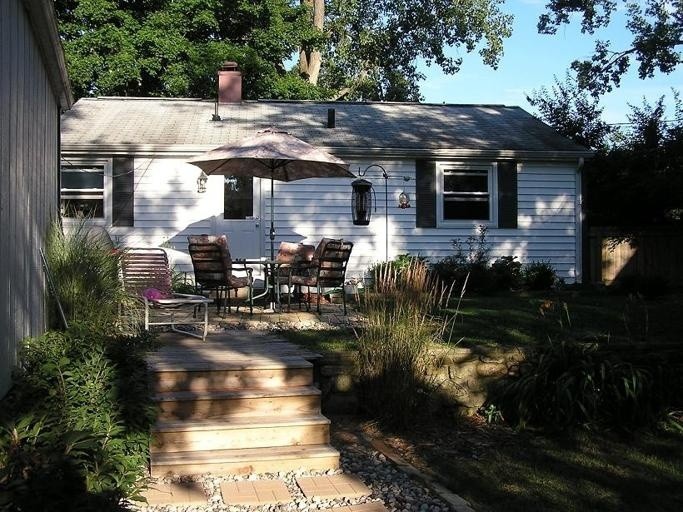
[350,164,389,261]
[197,170,208,193]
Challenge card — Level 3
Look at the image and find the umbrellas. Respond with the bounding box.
[187,123,358,300]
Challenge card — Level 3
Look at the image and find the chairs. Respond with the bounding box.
[115,246,214,342]
[185,233,353,318]
